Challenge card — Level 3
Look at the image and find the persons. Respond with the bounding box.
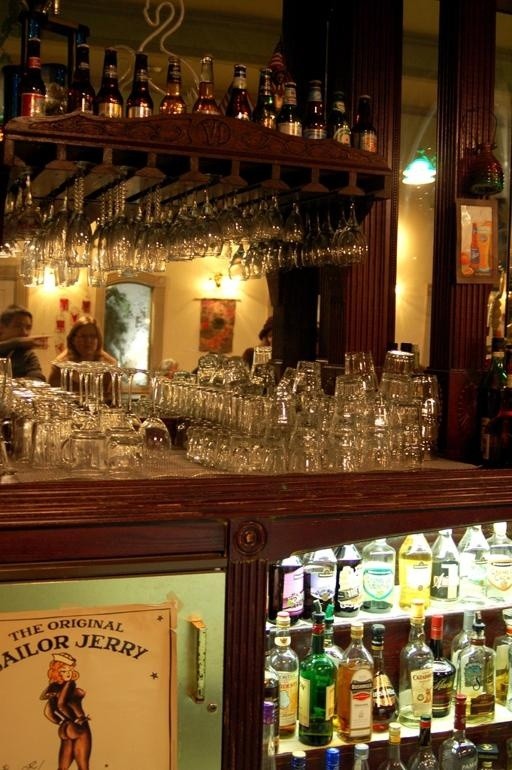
[48,316,118,391]
[41,653,92,770]
[159,358,180,379]
[242,316,273,366]
[0,304,53,382]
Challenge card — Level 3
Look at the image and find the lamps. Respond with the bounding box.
[402,147,436,185]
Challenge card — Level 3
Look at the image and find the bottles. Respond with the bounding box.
[291,750,306,770]
[427,614,456,718]
[262,701,276,770]
[486,522,512,604]
[192,54,224,117]
[494,608,512,707]
[506,644,512,713]
[253,68,278,131]
[476,337,511,467]
[408,714,441,770]
[303,80,329,141]
[457,525,491,609]
[301,548,338,622]
[398,533,433,612]
[352,94,378,154]
[336,622,374,742]
[476,743,498,770]
[385,722,406,770]
[334,543,363,618]
[66,31,96,116]
[324,616,345,669]
[126,52,153,119]
[351,743,370,770]
[369,624,400,733]
[266,554,304,626]
[225,63,253,123]
[265,612,299,739]
[399,600,434,729]
[430,528,460,610]
[298,612,337,747]
[159,56,187,116]
[450,609,477,704]
[439,695,479,770]
[95,47,124,121]
[361,538,397,613]
[456,618,497,724]
[278,82,304,137]
[329,91,353,151]
[17,16,46,118]
[263,630,280,753]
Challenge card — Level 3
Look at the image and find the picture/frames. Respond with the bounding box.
[456,197,498,284]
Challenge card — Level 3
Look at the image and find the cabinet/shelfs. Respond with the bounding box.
[0,452,227,769]
[223,459,512,770]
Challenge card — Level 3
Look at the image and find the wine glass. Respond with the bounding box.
[154,348,441,474]
[0,358,170,474]
[226,194,370,279]
[0,172,228,290]
